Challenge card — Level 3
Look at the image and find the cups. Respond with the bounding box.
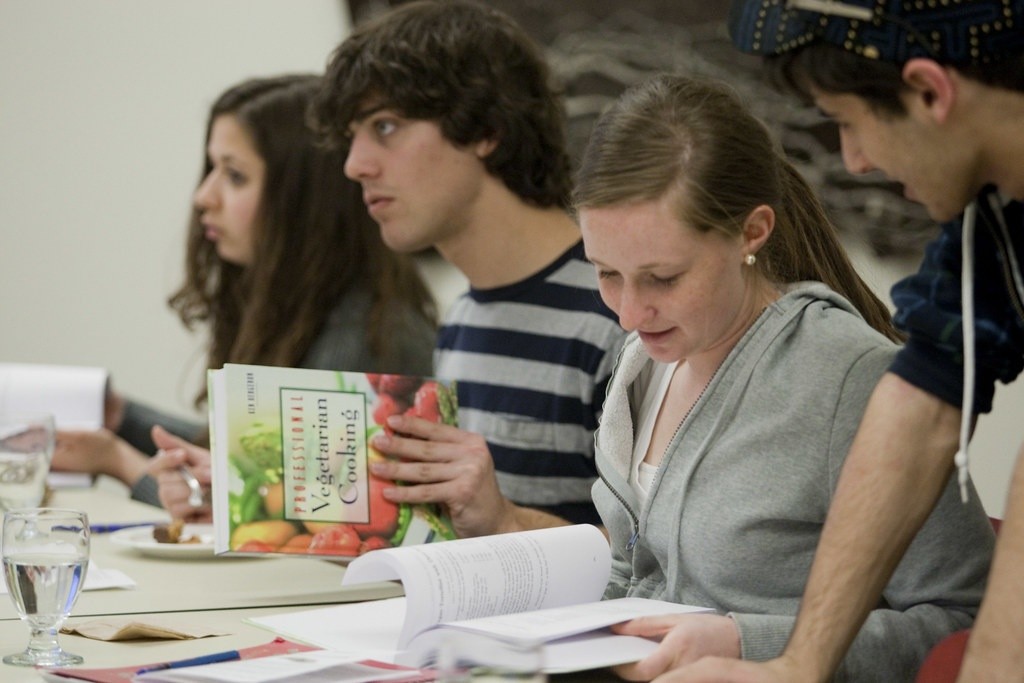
[0,411,55,510]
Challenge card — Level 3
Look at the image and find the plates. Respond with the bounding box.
[111,523,215,558]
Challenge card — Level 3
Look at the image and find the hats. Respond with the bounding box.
[725,0,1024,57]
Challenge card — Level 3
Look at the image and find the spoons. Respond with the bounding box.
[160,449,208,507]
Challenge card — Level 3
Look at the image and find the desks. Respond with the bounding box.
[1,473,545,683]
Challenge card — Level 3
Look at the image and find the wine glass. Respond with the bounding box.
[3,508,91,667]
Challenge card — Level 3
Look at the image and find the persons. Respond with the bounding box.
[653,0,1024,683]
[52,74,436,522]
[573,74,997,683]
[148,0,626,548]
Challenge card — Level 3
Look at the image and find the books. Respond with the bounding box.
[340,523,720,673]
[1,357,109,490]
[208,362,459,561]
